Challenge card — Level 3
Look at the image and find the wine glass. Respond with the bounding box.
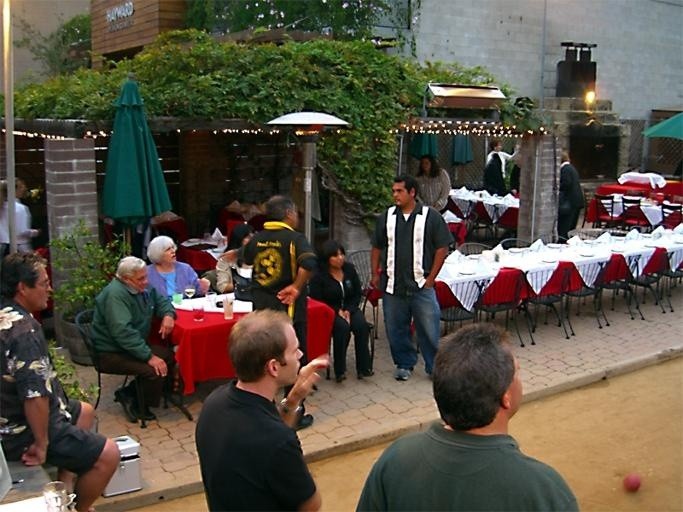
[184,284,196,307]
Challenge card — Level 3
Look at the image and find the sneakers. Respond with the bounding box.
[394,366,414,381]
[295,413,313,430]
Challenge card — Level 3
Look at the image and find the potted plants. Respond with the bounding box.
[42,216,131,369]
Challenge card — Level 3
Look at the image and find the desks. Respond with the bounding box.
[173,291,335,422]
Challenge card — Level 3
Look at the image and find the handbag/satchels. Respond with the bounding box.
[230,264,254,301]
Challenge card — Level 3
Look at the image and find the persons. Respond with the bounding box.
[88,256,176,422]
[195,310,331,512]
[1,176,41,255]
[485,140,523,193]
[558,152,583,241]
[353,322,580,511]
[1,254,120,512]
[141,197,268,303]
[309,239,374,382]
[241,195,314,431]
[369,175,454,380]
[416,155,450,213]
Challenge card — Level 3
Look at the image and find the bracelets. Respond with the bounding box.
[281,399,300,417]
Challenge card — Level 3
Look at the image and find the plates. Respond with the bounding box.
[188,238,201,243]
[605,192,683,212]
[450,230,682,277]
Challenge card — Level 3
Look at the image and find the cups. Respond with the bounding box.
[42,479,70,512]
[203,233,211,241]
[205,291,218,308]
[223,298,234,320]
[172,291,183,307]
[192,307,204,322]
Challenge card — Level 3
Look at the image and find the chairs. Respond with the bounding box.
[76,309,193,428]
[432,172,680,348]
[150,211,245,272]
[347,250,384,339]
[358,296,375,370]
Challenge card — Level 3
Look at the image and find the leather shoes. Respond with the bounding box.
[113,388,138,424]
[336,373,345,384]
[356,369,374,380]
[135,400,157,421]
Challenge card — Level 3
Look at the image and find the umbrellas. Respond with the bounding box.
[640,111,683,142]
[100,77,173,255]
